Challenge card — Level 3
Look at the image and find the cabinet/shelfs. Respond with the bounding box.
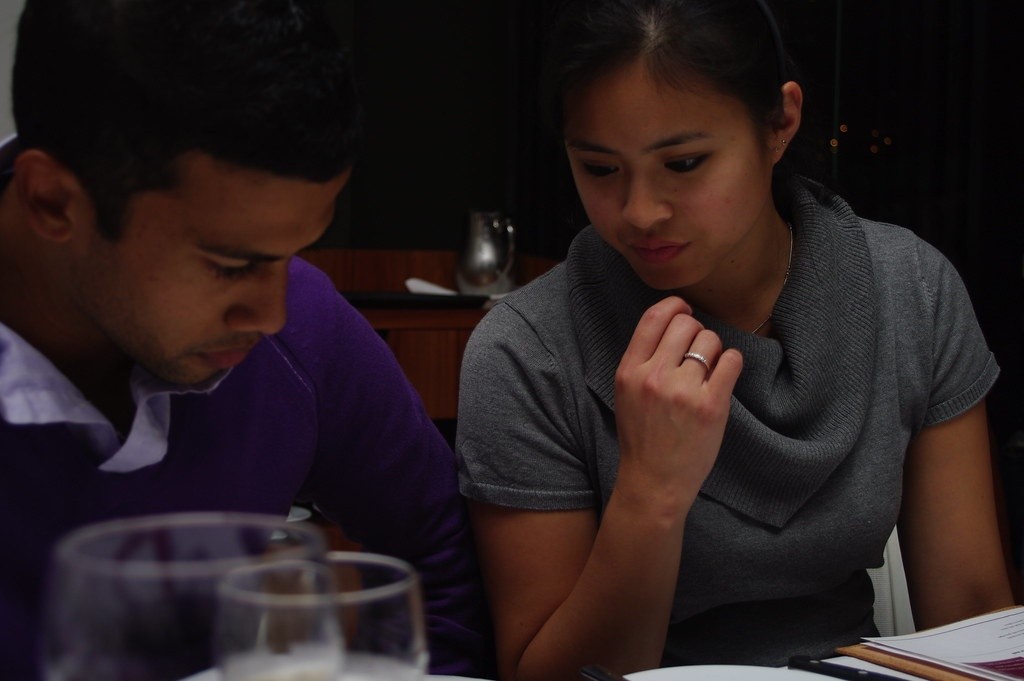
[300,247,564,419]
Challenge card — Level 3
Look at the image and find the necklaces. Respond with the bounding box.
[750,221,795,336]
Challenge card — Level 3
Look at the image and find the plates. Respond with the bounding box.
[625,664,846,681]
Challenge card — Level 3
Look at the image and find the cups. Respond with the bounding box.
[457,209,512,288]
[34,510,324,681]
[210,551,428,680]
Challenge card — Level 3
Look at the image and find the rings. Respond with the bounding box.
[683,351,712,373]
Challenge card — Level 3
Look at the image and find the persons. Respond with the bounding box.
[0,0,498,681]
[455,0,1012,681]
